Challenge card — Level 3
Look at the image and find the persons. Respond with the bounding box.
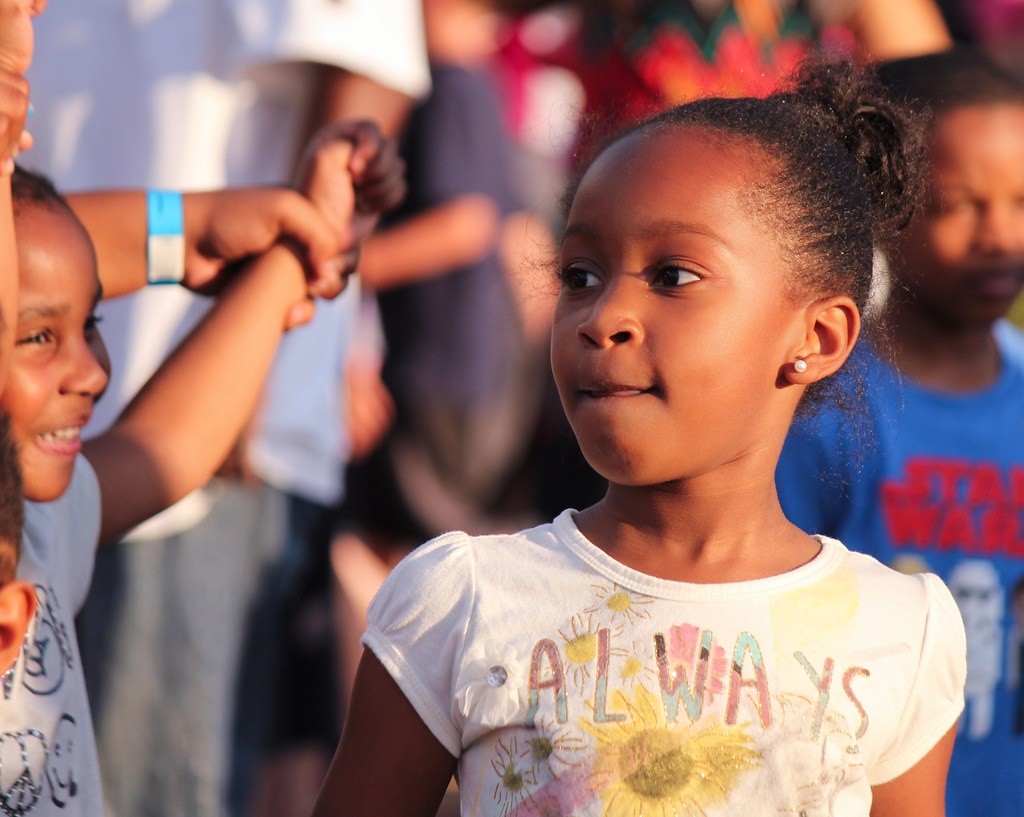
[0,2,431,817]
[326,51,510,730]
[1,68,344,329]
[306,56,967,817]
[769,56,1024,816]
[1,1,406,817]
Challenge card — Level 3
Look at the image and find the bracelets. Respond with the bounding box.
[145,186,185,285]
